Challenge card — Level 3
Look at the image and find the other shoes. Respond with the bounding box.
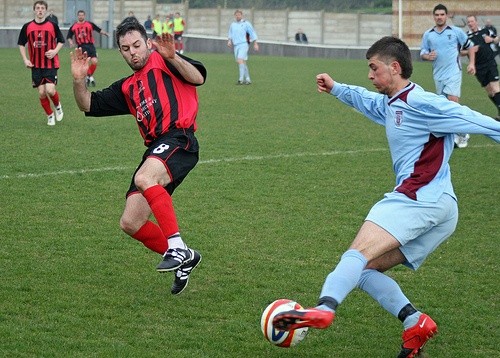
[86,75,96,87]
[452,133,472,149]
[176,49,184,54]
[236,80,251,85]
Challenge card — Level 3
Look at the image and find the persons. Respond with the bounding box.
[228,10,260,85]
[295,27,308,44]
[272,36,500,358]
[162,16,173,35]
[464,14,500,121]
[17,0,65,125]
[67,10,111,87]
[172,12,186,54]
[121,11,138,25]
[150,15,162,38]
[144,15,152,32]
[46,9,59,26]
[484,19,500,59]
[420,4,476,148]
[69,20,207,294]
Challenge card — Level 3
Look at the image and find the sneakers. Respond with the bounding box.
[54,103,63,121]
[47,112,55,126]
[398,313,437,357]
[155,243,193,274]
[272,307,337,331]
[171,248,202,296]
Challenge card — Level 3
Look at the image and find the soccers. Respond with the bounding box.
[261,299,308,348]
